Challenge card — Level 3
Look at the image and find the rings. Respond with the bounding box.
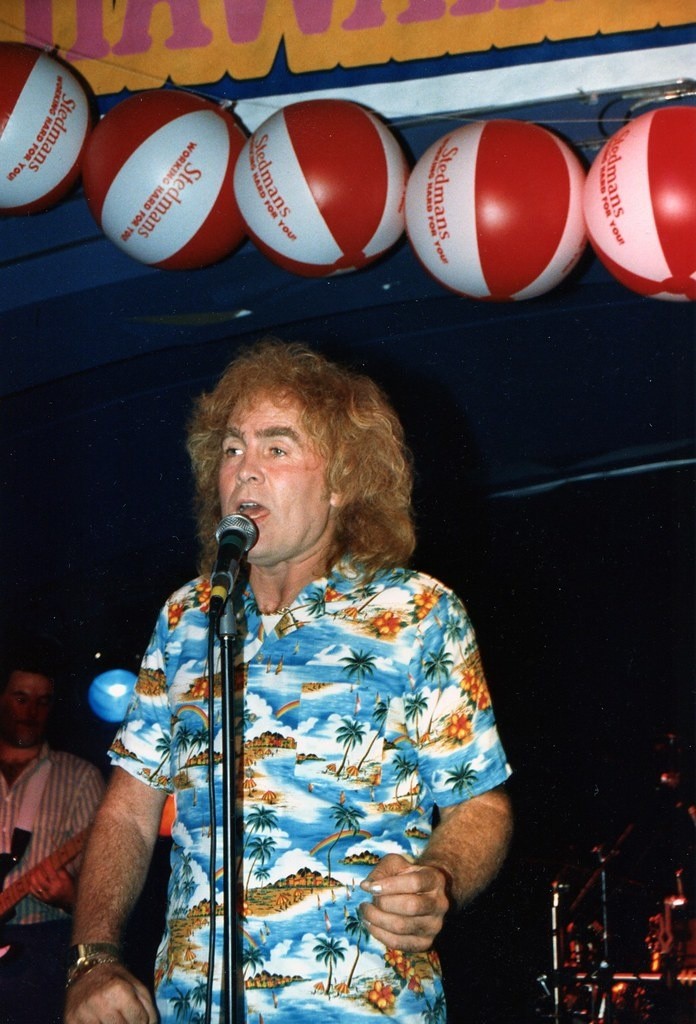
[37,887,45,894]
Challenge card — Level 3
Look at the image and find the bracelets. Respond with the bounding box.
[65,955,119,990]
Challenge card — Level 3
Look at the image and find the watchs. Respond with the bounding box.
[62,942,132,973]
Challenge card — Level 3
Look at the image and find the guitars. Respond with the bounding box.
[0,810,101,926]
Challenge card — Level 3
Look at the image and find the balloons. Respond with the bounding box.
[231,99,410,276]
[403,117,588,301]
[82,89,249,270]
[0,39,91,213]
[580,105,696,300]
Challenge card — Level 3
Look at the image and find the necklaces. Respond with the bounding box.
[260,607,290,615]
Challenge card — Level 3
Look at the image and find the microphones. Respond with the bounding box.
[207,514,257,620]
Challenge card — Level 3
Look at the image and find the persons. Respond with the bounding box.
[60,343,514,1024]
[0,653,106,1024]
